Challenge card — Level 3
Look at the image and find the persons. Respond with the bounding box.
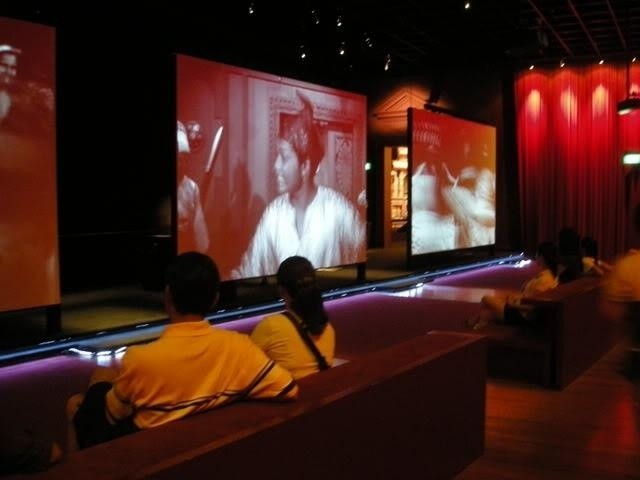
[464,224,613,333]
[1,44,22,120]
[248,258,337,381]
[177,120,211,252]
[61,249,299,456]
[227,103,366,283]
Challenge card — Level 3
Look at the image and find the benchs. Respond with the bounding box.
[480,267,629,390]
[0,330,488,480]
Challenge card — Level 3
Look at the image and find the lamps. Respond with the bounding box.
[617,98,640,116]
[299,9,391,70]
[464,0,470,9]
